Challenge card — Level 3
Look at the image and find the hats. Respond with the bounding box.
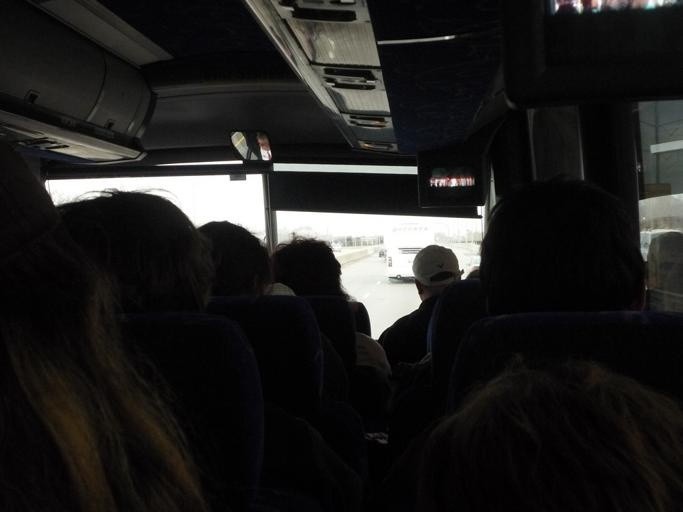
[412,245,464,288]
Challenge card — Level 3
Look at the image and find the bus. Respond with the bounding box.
[383,220,435,283]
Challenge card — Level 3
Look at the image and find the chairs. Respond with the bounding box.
[114,281,683,506]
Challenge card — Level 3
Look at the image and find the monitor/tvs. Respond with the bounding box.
[417,150,483,208]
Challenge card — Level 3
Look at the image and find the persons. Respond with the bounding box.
[1,142,392,512]
[380,172,683,512]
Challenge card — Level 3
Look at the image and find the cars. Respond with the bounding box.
[640,228,682,263]
